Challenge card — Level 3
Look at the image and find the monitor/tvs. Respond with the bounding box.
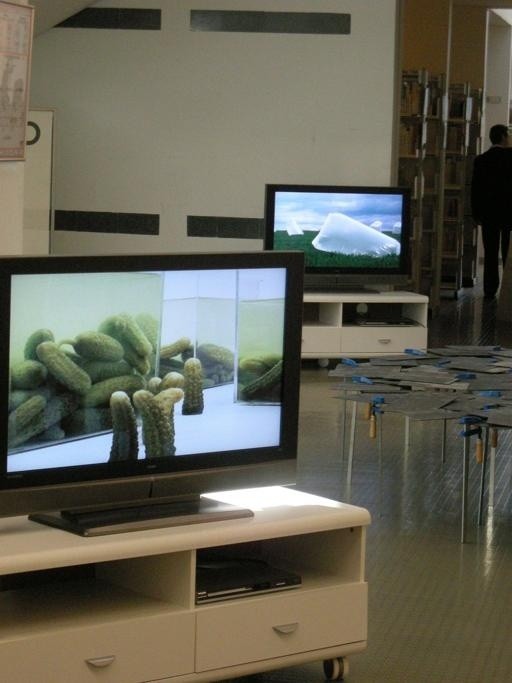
[264,184,412,294]
[0,249,306,537]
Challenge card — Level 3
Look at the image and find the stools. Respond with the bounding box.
[329,343,510,544]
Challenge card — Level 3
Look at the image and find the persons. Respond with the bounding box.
[469,125,512,299]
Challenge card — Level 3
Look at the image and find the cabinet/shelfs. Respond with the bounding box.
[300,289,428,366]
[401,67,474,319]
[1,484,371,682]
